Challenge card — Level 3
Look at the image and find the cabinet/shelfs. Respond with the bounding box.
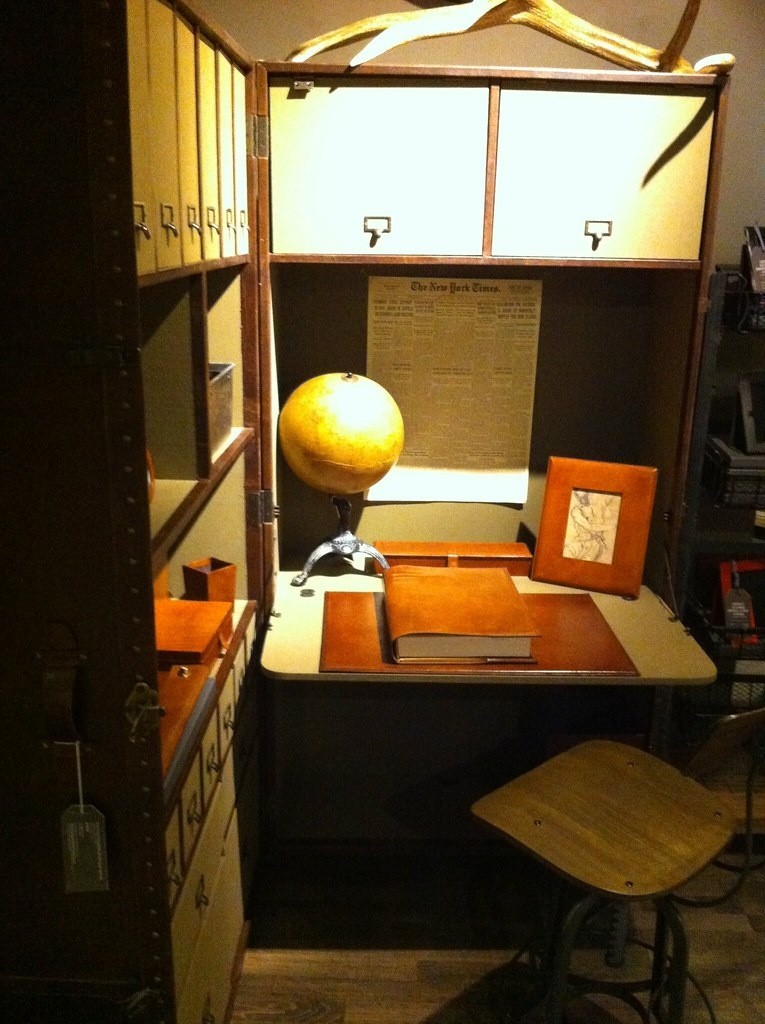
[0,1,731,1024]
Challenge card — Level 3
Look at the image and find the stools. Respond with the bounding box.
[466,739,736,1024]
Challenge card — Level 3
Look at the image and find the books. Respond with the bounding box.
[384,565,544,668]
